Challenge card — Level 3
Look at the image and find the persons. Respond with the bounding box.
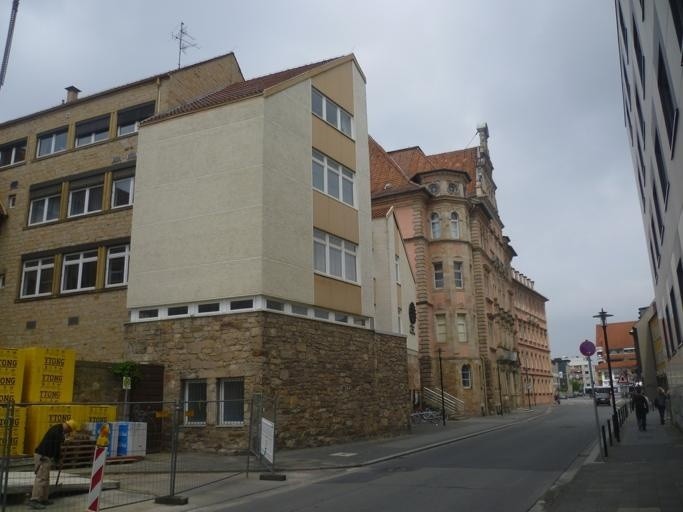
[30,420,78,509]
[655,387,667,425]
[632,389,649,431]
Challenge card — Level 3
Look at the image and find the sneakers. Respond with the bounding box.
[29,499,53,509]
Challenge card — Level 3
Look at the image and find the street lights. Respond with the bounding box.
[435,347,446,426]
[592,307,621,442]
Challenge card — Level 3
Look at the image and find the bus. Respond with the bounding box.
[595,386,612,406]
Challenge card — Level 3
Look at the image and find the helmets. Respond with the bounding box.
[65,419,78,429]
